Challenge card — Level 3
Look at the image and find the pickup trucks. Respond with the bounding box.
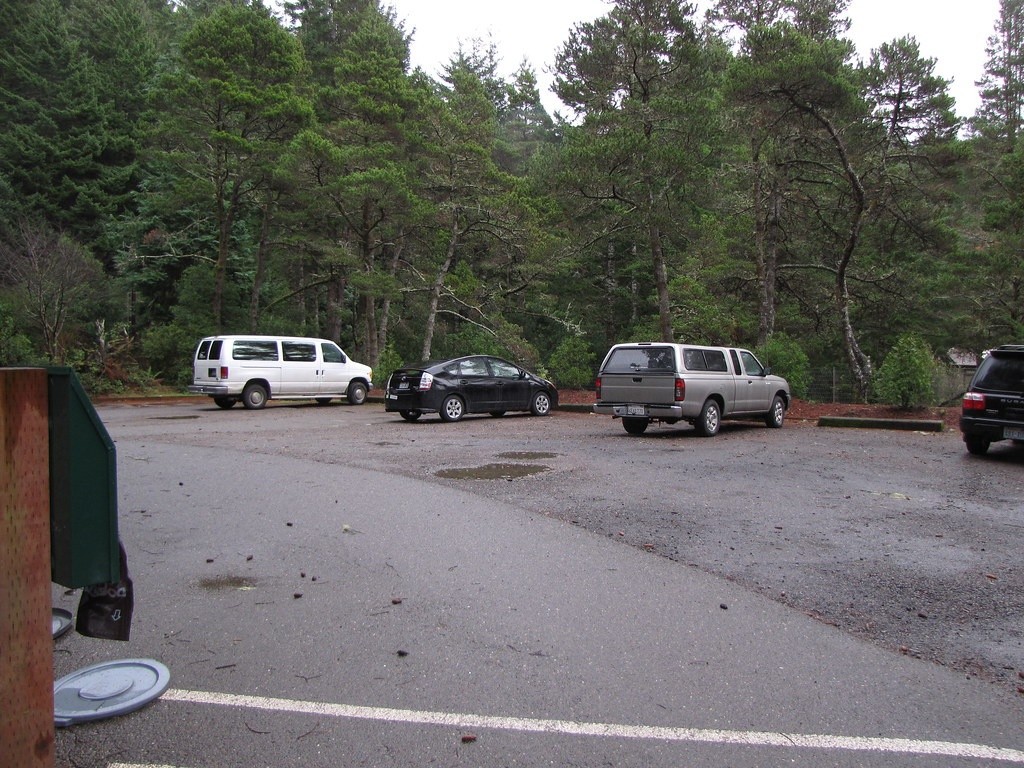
[593,342,791,437]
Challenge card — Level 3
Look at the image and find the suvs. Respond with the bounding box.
[958,346,1024,454]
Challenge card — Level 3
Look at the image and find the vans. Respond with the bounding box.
[189,335,373,410]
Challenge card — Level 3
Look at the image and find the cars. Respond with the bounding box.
[385,355,558,422]
